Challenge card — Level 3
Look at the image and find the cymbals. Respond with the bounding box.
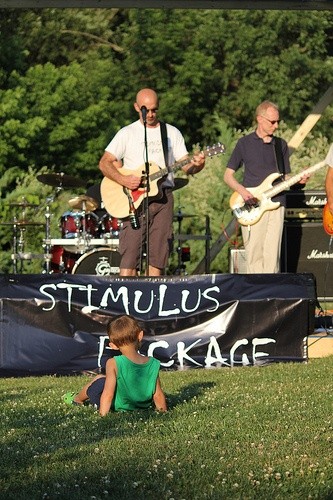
[0,220,44,227]
[36,172,86,188]
[4,202,39,207]
[68,194,99,211]
[173,212,197,218]
[172,178,189,192]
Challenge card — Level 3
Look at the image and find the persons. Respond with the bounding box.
[63,313,167,417]
[85,159,122,220]
[325,143,333,215]
[224,101,312,274]
[98,87,206,278]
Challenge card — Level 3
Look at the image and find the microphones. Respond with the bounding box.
[141,106,147,123]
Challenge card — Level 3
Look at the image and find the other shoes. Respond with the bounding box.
[64,392,78,407]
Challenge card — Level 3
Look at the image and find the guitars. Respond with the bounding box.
[323,203,333,236]
[229,161,326,226]
[100,142,225,219]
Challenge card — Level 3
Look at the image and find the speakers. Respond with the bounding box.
[280,221,333,301]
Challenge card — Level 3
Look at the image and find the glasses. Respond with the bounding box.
[265,118,281,124]
[138,105,158,112]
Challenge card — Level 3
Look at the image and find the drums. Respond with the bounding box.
[59,211,101,253]
[46,243,79,274]
[71,247,121,275]
[99,213,124,238]
